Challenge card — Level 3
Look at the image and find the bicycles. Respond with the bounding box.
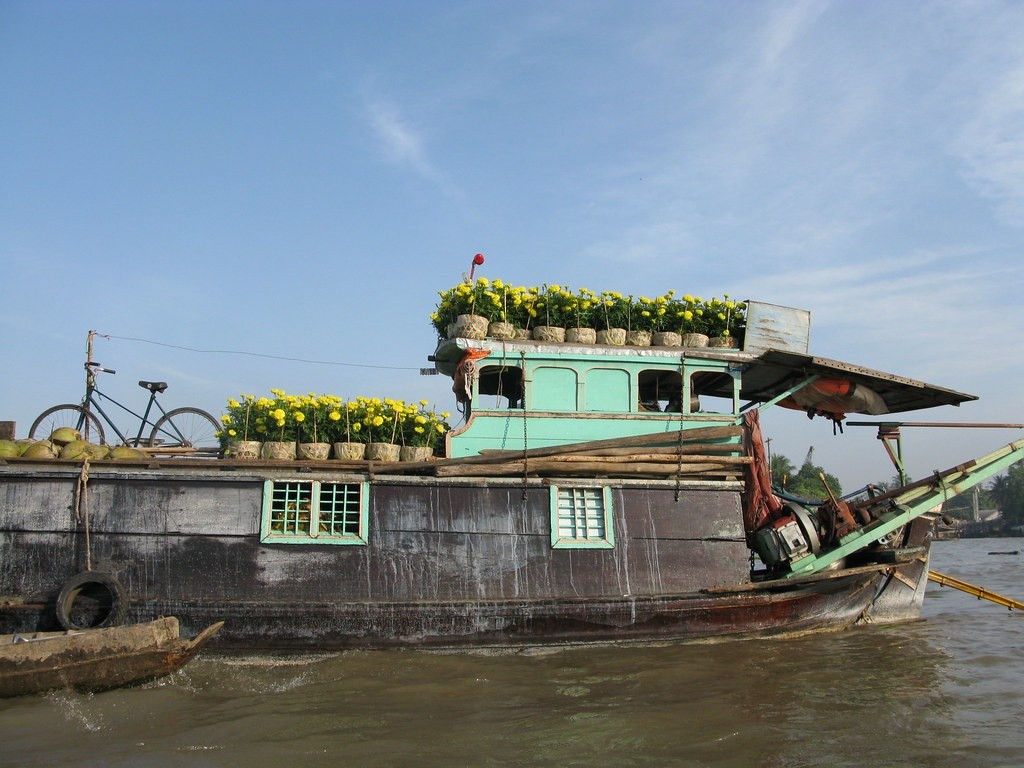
[28,361,225,459]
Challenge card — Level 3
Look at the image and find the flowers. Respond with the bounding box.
[428,276,746,336]
[215,388,451,456]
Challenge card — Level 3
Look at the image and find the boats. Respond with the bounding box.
[0,254,1023,654]
[0,615,225,699]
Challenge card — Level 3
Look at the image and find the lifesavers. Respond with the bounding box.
[790,377,888,416]
[55,570,129,632]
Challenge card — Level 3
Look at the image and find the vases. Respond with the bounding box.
[626,330,653,347]
[488,322,516,342]
[400,446,433,462]
[227,440,261,461]
[710,337,739,350]
[533,326,566,344]
[333,442,367,461]
[456,314,490,340]
[652,332,682,347]
[446,323,456,338]
[515,329,533,340]
[565,328,597,345]
[299,443,331,461]
[259,442,296,461]
[366,443,401,462]
[681,333,710,348]
[596,327,627,346]
